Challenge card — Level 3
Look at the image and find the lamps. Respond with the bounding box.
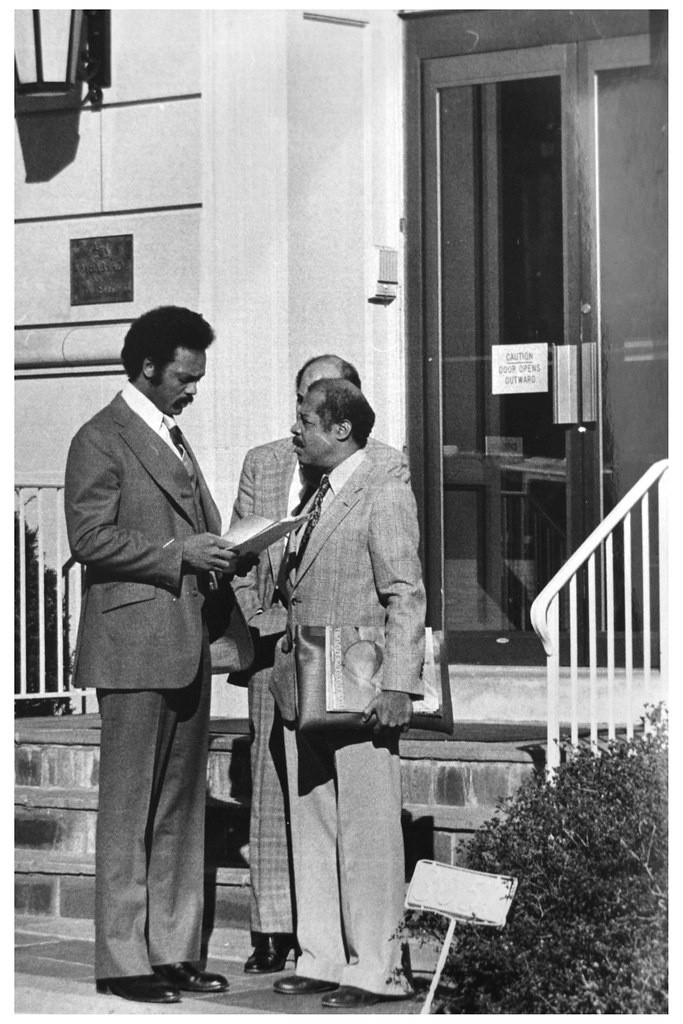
[14,9,112,105]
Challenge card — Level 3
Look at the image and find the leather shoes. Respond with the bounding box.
[151,962,230,991]
[244,934,296,973]
[272,976,339,994]
[321,986,384,1007]
[96,975,183,1003]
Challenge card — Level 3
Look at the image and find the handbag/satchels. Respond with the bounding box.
[296,624,454,737]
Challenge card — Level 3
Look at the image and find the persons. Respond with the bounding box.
[228,355,427,1008]
[66,306,259,1004]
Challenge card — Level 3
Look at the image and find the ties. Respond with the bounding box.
[297,476,330,573]
[163,414,183,454]
